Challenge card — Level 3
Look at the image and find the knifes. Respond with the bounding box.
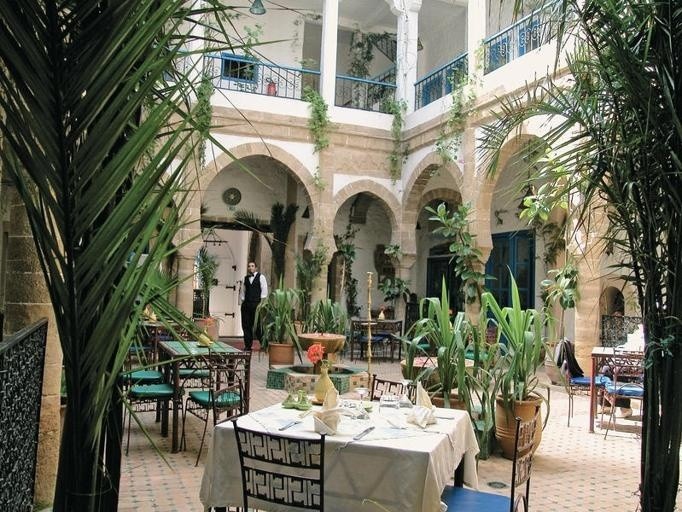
[353,426,375,442]
[279,421,301,432]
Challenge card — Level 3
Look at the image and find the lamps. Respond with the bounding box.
[249,0,270,18]
[518,138,539,210]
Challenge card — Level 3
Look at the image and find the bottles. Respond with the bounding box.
[379,395,401,420]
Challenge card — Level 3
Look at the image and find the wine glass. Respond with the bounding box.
[351,386,369,422]
[400,378,413,413]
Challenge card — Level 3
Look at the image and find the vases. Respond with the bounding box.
[192,244,226,341]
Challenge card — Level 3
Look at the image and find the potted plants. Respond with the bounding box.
[389,274,472,411]
[298,296,348,374]
[252,270,308,369]
[473,263,574,461]
[534,261,581,385]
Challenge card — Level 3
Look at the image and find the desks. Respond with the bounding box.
[158,339,250,454]
[590,347,643,434]
[214,397,468,512]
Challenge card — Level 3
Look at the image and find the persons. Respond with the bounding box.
[237,261,269,352]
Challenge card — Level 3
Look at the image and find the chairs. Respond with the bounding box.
[562,337,643,441]
[345,309,503,389]
[211,372,541,512]
[123,307,252,467]
